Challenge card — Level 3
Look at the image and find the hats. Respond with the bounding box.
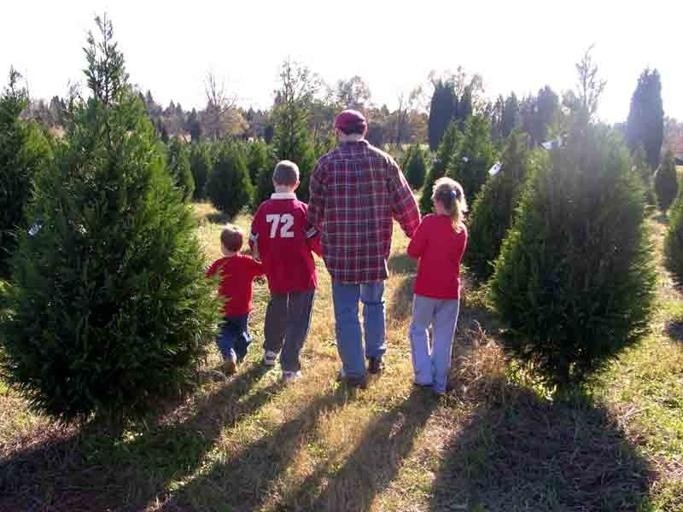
[334,109,367,129]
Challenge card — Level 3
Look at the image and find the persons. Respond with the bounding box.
[306,110,422,388]
[247,160,322,384]
[205,226,266,376]
[408,176,469,396]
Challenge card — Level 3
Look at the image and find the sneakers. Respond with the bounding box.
[223,358,236,370]
[338,368,365,389]
[368,357,381,374]
[264,351,276,366]
[282,371,301,384]
